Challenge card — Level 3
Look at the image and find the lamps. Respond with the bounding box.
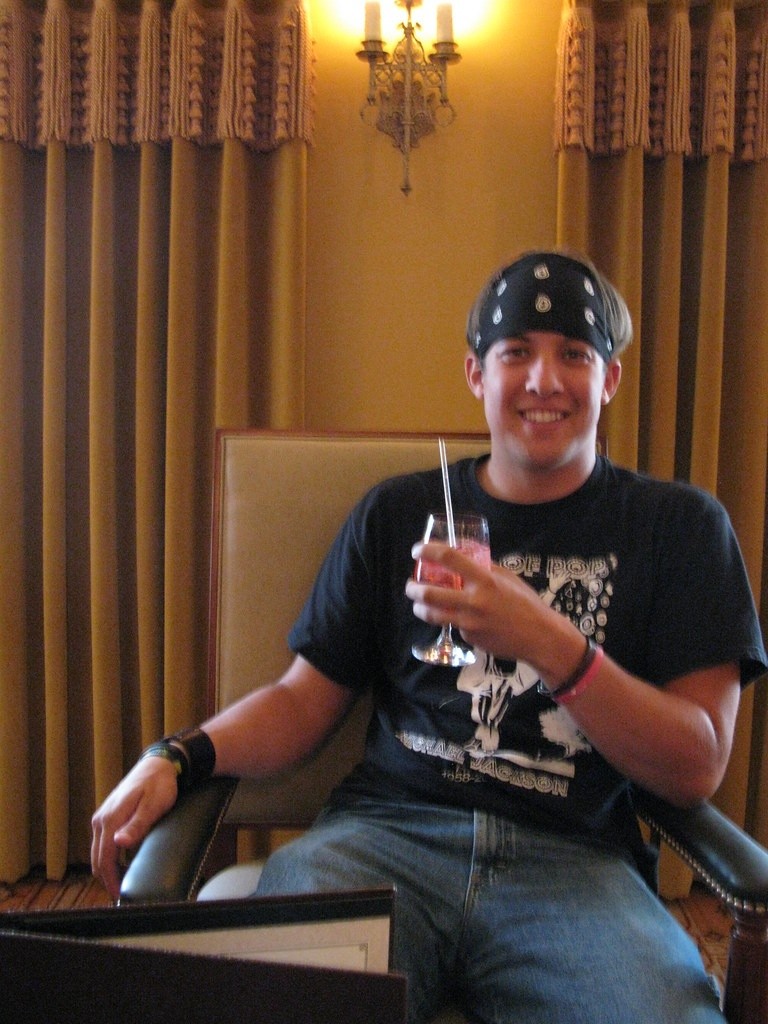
[355,0,463,201]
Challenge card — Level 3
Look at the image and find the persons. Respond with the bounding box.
[91,249,767,1024]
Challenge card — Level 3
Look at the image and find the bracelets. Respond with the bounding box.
[546,634,604,705]
[141,717,215,797]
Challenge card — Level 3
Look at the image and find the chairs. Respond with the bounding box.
[121,427,767,1024]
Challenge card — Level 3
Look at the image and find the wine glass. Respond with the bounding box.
[412,513,492,666]
